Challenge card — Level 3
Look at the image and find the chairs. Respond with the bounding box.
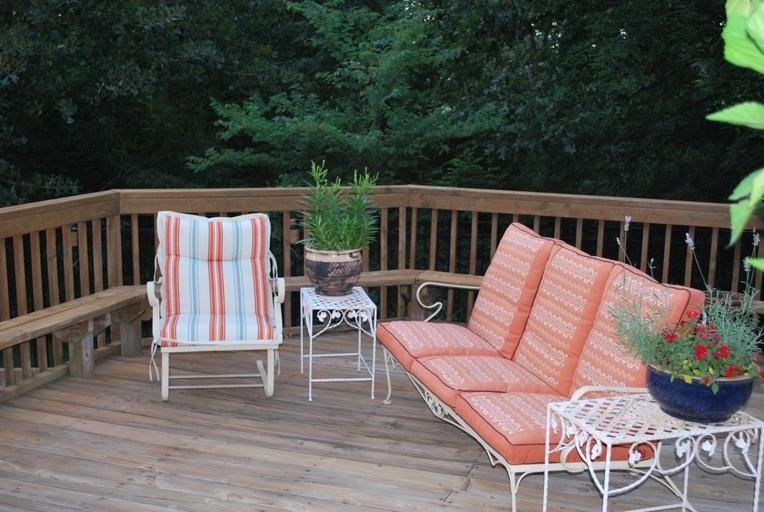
[146,211,285,402]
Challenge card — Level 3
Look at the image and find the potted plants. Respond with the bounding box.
[291,160,380,296]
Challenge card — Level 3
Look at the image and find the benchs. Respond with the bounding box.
[416,270,484,321]
[376,222,706,512]
[285,269,425,321]
[1,285,146,378]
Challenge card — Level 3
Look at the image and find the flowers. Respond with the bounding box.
[607,214,764,395]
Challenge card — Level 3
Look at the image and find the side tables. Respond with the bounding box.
[300,287,378,402]
[543,392,764,512]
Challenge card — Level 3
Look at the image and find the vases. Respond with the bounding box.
[646,362,756,423]
